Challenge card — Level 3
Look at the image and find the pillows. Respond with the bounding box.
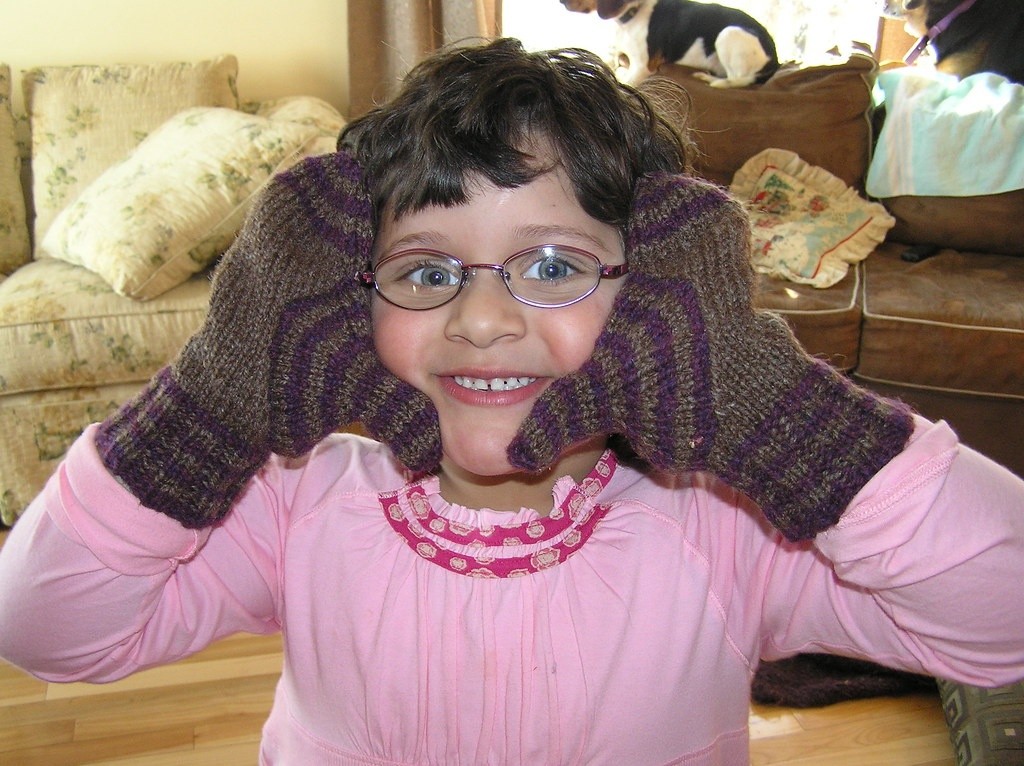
[21,53,240,262]
[39,108,322,302]
[865,58,1024,258]
[653,38,880,204]
[0,64,31,277]
[935,675,1024,766]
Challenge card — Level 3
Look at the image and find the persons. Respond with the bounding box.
[0,38,1024,766]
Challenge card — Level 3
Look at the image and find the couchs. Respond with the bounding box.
[0,94,352,528]
[637,39,1024,482]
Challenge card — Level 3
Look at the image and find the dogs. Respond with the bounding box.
[559,0,781,89]
[876,0,1024,86]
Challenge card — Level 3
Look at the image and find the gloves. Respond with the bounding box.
[93,153,443,529]
[505,171,913,543]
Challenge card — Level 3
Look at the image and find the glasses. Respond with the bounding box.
[353,243,628,309]
